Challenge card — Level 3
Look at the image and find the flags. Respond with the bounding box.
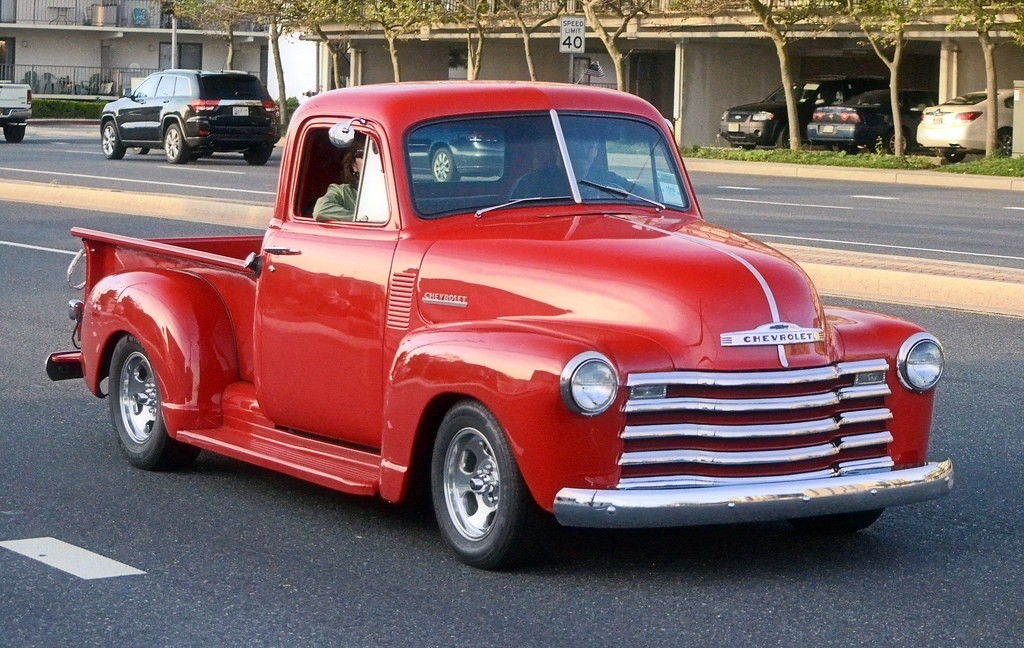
[585,61,605,77]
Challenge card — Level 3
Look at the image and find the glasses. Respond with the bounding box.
[354,151,364,159]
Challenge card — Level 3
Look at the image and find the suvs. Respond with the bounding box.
[99,68,280,167]
[718,74,888,150]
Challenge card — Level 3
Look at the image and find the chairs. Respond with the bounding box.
[829,91,843,105]
[20,71,103,94]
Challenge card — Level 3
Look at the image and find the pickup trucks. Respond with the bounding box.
[0,77,35,144]
[46,79,955,573]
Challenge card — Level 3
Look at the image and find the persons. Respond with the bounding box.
[312,135,366,222]
[508,133,658,204]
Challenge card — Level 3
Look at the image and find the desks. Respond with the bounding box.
[47,5,76,25]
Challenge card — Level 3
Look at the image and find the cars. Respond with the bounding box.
[806,88,938,156]
[914,88,1014,161]
